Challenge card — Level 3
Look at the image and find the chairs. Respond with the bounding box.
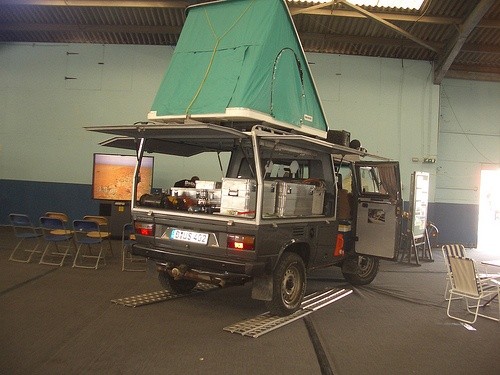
[8,212,152,272]
[335,173,354,220]
[441,244,500,325]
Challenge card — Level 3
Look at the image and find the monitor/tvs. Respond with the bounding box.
[92,153,154,201]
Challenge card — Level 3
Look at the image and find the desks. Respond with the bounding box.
[481,260,500,274]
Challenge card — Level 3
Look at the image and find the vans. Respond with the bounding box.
[82,119,405,317]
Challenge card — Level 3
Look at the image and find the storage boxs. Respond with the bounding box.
[325,130,351,147]
[220,177,278,219]
[171,181,222,205]
[275,179,328,219]
